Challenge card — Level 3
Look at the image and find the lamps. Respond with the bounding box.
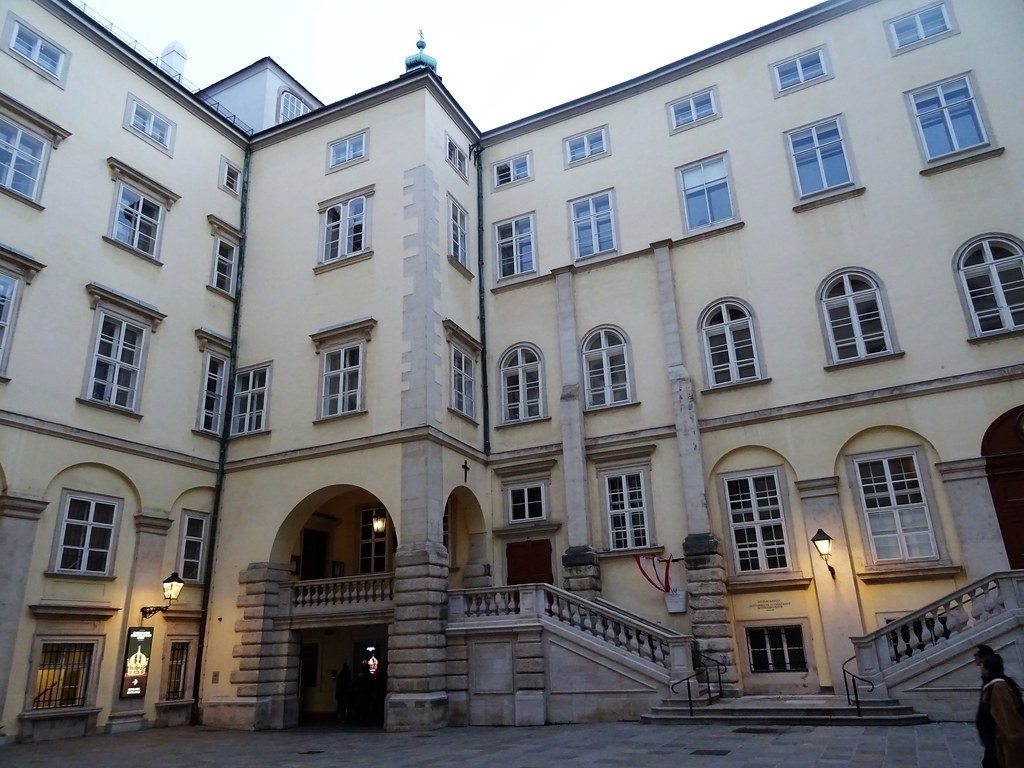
[140,572,186,619]
[370,509,387,536]
[811,528,836,579]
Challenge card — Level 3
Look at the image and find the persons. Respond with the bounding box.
[335,662,384,728]
[972,644,1004,767]
[979,653,1024,768]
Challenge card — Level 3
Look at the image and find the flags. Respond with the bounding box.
[635,554,674,592]
